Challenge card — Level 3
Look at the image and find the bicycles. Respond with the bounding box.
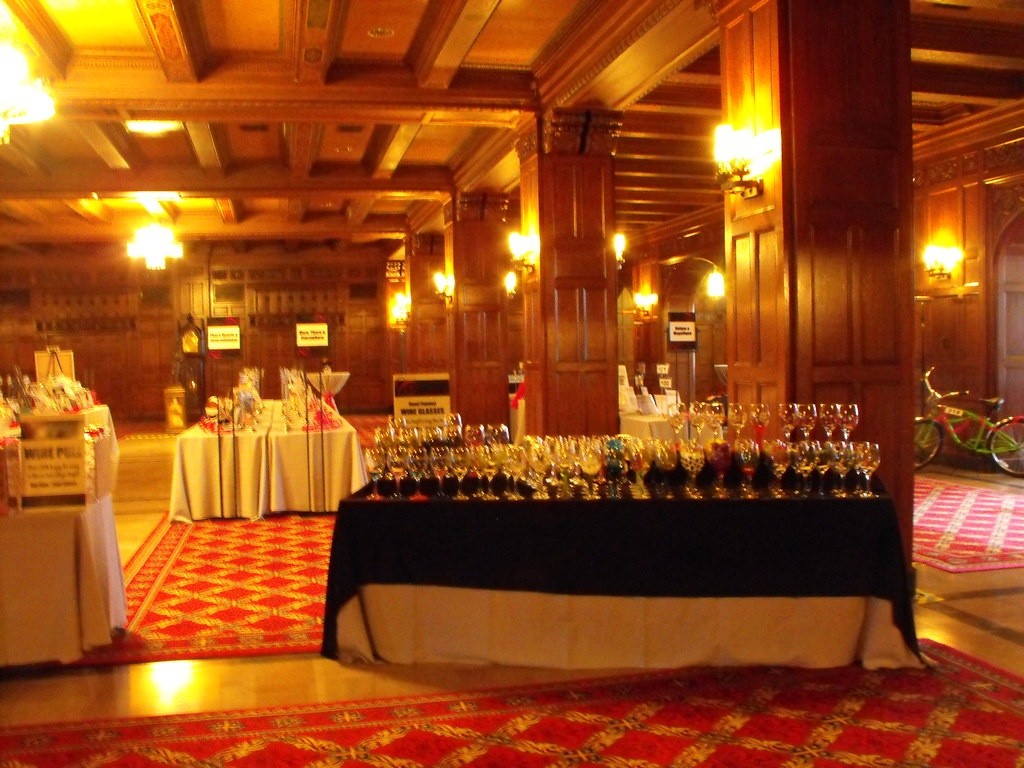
[914,364,1024,479]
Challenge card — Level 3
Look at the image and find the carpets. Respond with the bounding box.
[0,637,1024,768]
[911,472,1024,573]
[58,508,338,668]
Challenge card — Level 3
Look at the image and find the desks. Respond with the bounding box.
[170,398,371,522]
[321,453,940,673]
[0,494,127,671]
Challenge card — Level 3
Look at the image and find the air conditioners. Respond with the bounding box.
[507,227,535,275]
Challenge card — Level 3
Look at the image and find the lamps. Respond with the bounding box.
[712,124,762,201]
[922,242,963,280]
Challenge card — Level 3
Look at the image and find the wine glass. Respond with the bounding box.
[365,401,881,499]
